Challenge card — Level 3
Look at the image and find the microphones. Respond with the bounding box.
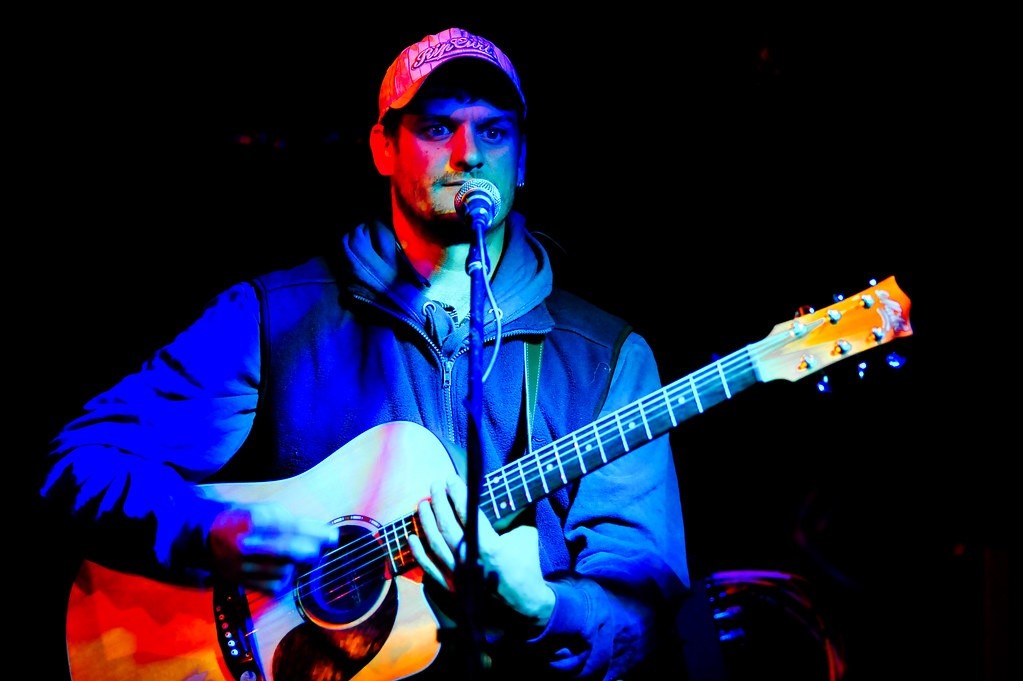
[454,176,501,226]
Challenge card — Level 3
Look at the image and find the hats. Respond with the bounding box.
[378,28,527,123]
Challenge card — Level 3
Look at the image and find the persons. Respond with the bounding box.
[22,28,691,680]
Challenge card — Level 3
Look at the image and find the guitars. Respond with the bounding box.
[62,269,915,681]
